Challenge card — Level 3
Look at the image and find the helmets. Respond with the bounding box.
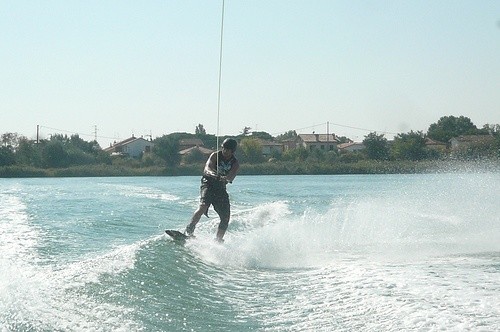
[221,138,237,151]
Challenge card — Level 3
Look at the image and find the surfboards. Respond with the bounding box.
[165,230,188,241]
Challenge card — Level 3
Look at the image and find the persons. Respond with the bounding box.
[181,138,240,245]
